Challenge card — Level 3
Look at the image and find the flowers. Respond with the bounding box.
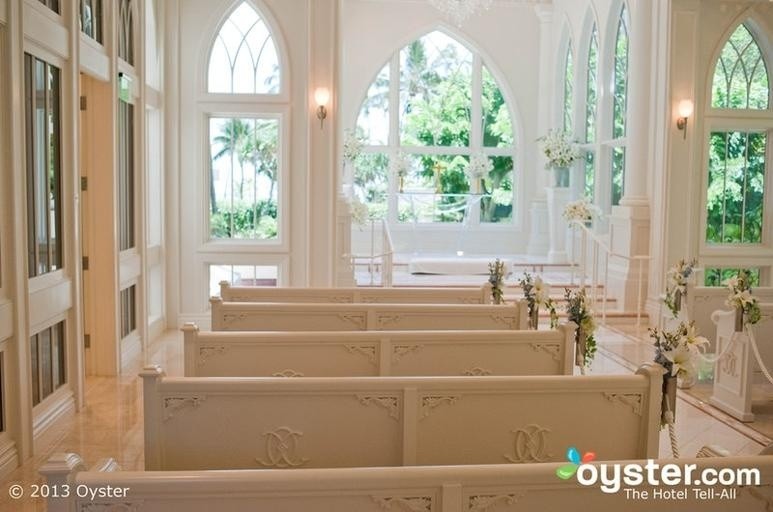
[346,194,370,231]
[342,127,364,163]
[463,152,494,180]
[724,267,761,332]
[662,258,699,319]
[563,281,597,371]
[486,257,559,330]
[392,152,412,177]
[647,319,710,432]
[533,126,588,171]
[560,196,605,231]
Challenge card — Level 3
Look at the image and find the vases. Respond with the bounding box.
[475,181,480,194]
[552,166,569,187]
[399,178,404,193]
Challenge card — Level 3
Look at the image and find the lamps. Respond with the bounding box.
[314,87,329,131]
[676,99,693,141]
